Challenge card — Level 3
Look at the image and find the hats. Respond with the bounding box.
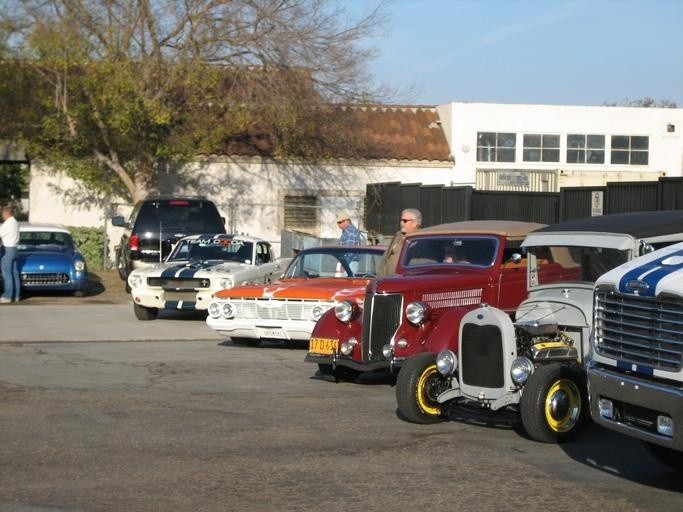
[336,214,352,221]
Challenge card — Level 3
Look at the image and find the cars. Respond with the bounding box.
[304,220,582,383]
[395,211,683,444]
[0,225,84,298]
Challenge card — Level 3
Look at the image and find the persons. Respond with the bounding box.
[0,207,21,304]
[375,208,423,279]
[335,210,364,278]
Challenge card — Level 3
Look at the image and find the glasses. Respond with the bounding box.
[336,220,345,224]
[400,218,412,223]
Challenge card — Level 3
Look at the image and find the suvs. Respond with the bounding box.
[588,237,683,458]
[115,198,227,292]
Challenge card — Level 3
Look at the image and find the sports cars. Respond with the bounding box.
[127,233,292,320]
[206,247,388,343]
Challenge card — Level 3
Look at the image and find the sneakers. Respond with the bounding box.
[0,295,19,305]
